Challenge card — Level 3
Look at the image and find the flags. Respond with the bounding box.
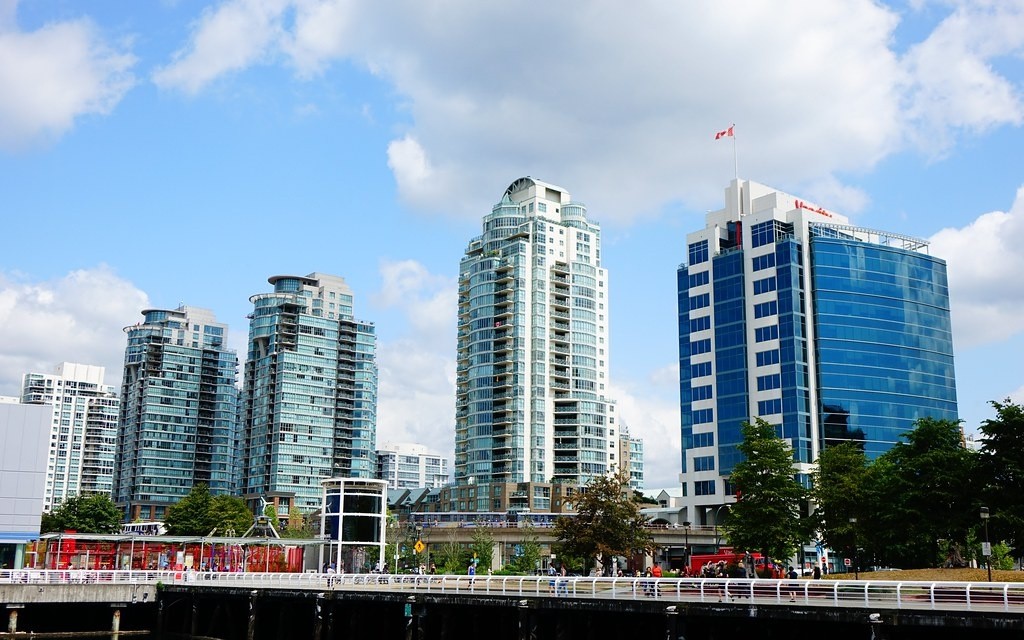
[715,126,734,140]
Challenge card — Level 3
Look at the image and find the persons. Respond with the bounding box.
[547,561,569,597]
[631,568,641,591]
[644,562,662,598]
[323,560,335,587]
[642,520,681,531]
[736,561,760,599]
[467,561,477,591]
[374,560,389,584]
[66,560,243,580]
[772,561,787,585]
[595,564,624,579]
[785,566,799,602]
[700,560,734,602]
[279,519,288,533]
[810,561,827,586]
[693,569,700,588]
[224,524,236,537]
[684,561,691,583]
[430,563,436,575]
[408,515,558,530]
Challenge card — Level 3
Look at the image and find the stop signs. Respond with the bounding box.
[821,556,825,563]
[844,559,850,565]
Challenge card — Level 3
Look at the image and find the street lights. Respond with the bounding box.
[715,505,731,550]
[979,506,993,590]
[849,513,857,579]
[683,521,690,573]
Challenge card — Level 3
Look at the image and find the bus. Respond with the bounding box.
[408,512,579,527]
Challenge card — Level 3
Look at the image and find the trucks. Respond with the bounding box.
[689,552,785,579]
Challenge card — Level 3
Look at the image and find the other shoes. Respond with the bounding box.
[791,598,793,600]
[790,600,796,602]
